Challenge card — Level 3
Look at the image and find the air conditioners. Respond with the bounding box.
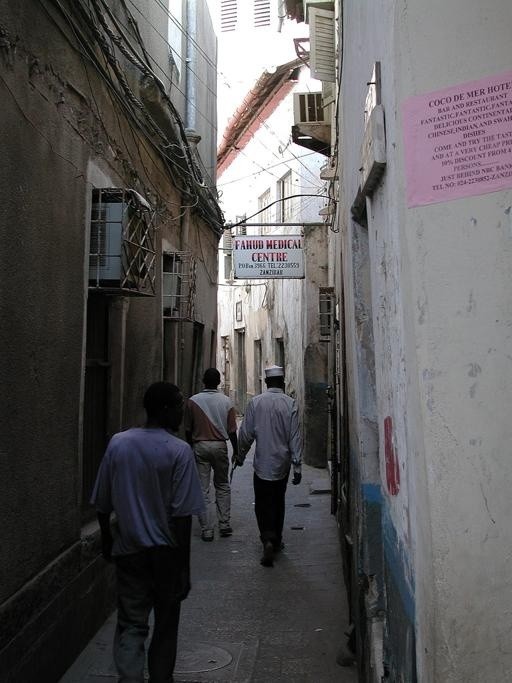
[292,90,332,125]
[162,271,182,312]
[88,201,143,287]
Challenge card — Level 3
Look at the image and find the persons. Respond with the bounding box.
[236,364,303,567]
[90,378,208,682]
[183,365,242,541]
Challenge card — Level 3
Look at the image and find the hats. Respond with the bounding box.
[263,364,284,378]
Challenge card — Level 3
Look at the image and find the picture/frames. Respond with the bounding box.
[236,299,242,322]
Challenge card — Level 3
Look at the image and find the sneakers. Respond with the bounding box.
[201,533,215,542]
[219,526,233,536]
[258,539,286,569]
